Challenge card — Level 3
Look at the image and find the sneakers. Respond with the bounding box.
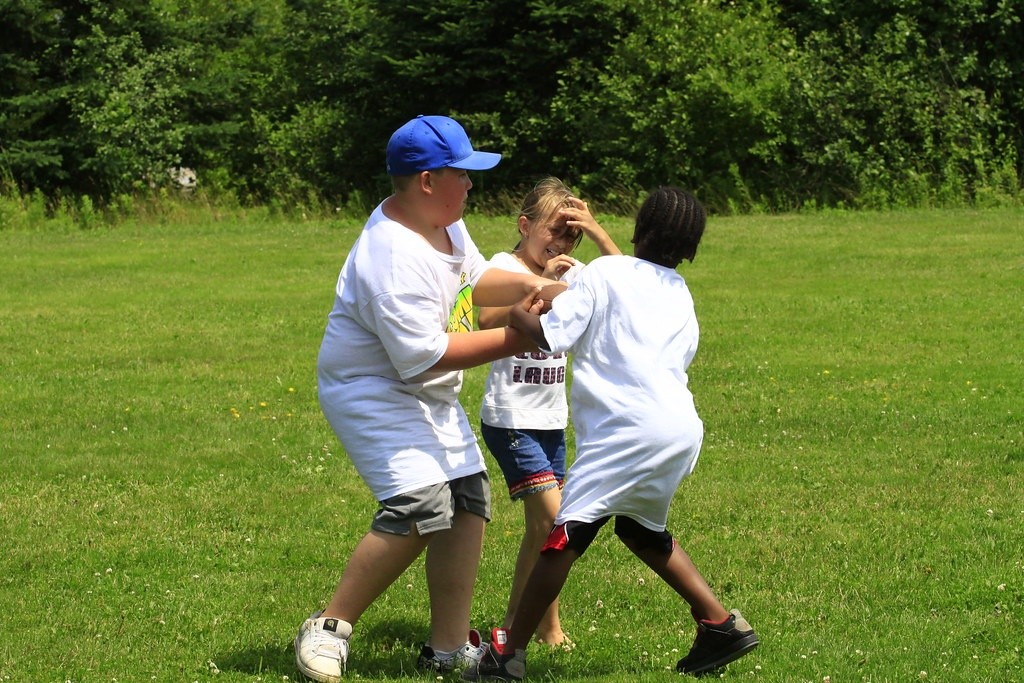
[416,629,490,673]
[461,628,529,682]
[294,609,353,683]
[676,607,759,676]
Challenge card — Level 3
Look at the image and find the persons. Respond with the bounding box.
[478,176,624,655]
[296,115,568,683]
[461,186,759,683]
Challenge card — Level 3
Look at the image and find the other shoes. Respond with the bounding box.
[534,632,576,655]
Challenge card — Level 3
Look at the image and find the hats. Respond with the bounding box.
[386,115,502,176]
